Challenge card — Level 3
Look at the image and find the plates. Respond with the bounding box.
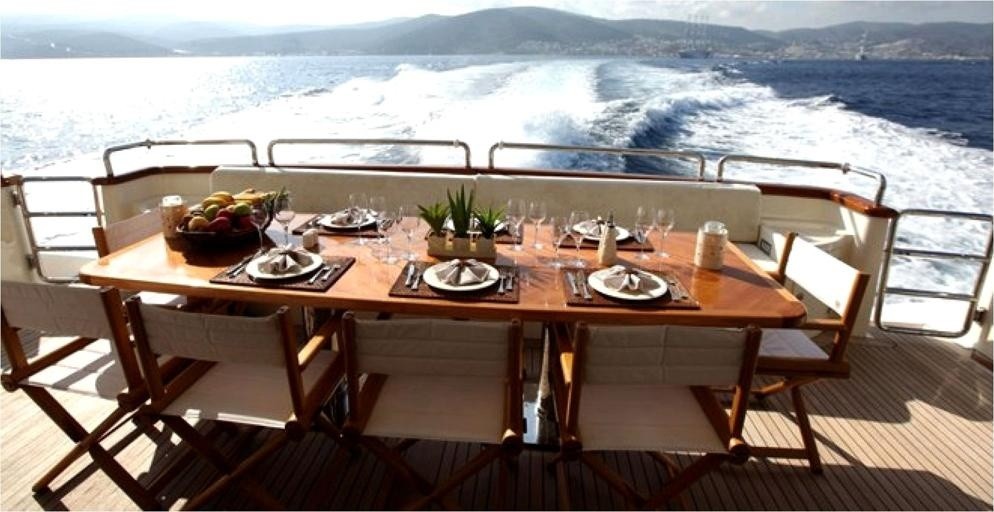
[421,259,499,291]
[587,265,668,300]
[572,219,630,242]
[246,249,321,277]
[319,211,376,230]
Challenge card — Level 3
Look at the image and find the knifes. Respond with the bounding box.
[226,248,256,278]
[665,274,691,302]
[497,267,516,294]
[565,268,592,301]
[309,259,341,283]
[401,261,424,290]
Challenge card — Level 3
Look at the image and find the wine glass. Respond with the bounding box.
[348,191,421,265]
[568,207,592,267]
[547,213,566,269]
[654,206,679,261]
[632,203,654,261]
[268,194,296,248]
[500,192,524,253]
[526,197,545,250]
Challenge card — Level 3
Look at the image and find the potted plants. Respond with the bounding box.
[417,184,508,258]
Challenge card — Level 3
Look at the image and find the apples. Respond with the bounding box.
[180,203,265,232]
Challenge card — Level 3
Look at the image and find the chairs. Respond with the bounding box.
[123,293,363,487]
[558,317,758,458]
[1,280,195,511]
[727,234,869,476]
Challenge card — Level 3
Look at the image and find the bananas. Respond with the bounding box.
[202,188,270,209]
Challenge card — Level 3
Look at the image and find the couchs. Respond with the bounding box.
[209,164,787,387]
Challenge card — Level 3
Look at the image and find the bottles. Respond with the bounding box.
[692,218,729,273]
